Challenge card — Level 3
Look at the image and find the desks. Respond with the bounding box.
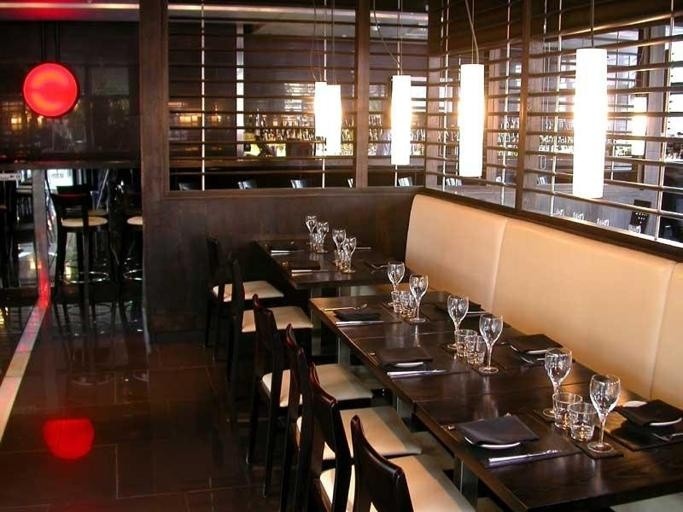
[307,291,509,363]
[414,383,683,512]
[337,314,607,431]
[255,240,421,356]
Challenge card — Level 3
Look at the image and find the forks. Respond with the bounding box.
[321,303,369,314]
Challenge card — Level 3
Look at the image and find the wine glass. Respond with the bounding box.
[306,217,359,275]
[479,311,505,376]
[407,273,428,325]
[446,295,469,349]
[541,349,573,418]
[385,259,405,307]
[587,373,620,454]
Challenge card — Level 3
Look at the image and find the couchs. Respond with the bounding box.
[405,193,683,409]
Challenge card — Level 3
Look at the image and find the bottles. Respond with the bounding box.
[253,107,315,139]
[369,115,380,140]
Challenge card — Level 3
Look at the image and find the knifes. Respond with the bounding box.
[382,368,473,380]
[336,321,403,327]
[292,267,335,275]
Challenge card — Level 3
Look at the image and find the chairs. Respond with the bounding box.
[351,415,413,512]
[279,324,422,512]
[291,362,423,512]
[0,167,143,320]
[225,258,316,400]
[204,237,284,361]
[246,294,373,497]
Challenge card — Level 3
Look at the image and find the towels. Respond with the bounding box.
[507,334,563,354]
[375,346,433,367]
[614,399,683,427]
[435,298,481,314]
[270,242,299,250]
[333,306,381,321]
[456,414,540,446]
[287,260,321,270]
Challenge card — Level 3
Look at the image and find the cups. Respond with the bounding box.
[567,402,597,445]
[572,211,584,220]
[464,335,488,365]
[390,290,406,313]
[628,224,641,233]
[551,392,584,432]
[554,208,565,216]
[454,328,475,359]
[400,292,417,319]
